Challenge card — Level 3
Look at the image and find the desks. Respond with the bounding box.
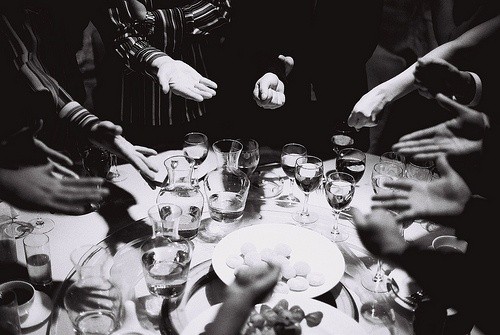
[0,139,483,335]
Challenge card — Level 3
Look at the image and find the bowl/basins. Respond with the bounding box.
[0,281,35,317]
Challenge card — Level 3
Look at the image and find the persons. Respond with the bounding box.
[208,259,281,335]
[252,0,500,334]
[0,1,279,216]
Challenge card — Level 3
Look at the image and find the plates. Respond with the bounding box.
[211,222,346,299]
[18,289,54,329]
[180,294,366,335]
[385,266,458,316]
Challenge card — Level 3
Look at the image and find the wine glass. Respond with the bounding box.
[82,145,112,210]
[334,147,366,219]
[229,137,260,201]
[371,151,435,222]
[360,209,405,294]
[182,132,209,189]
[324,171,356,243]
[276,143,308,204]
[291,155,324,224]
[28,209,56,234]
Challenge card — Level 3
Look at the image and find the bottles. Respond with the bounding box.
[141,203,194,299]
[155,155,204,244]
[62,242,123,335]
[203,139,250,223]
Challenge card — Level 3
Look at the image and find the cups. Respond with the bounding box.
[330,122,356,156]
[0,289,22,335]
[22,232,52,287]
[432,235,468,252]
